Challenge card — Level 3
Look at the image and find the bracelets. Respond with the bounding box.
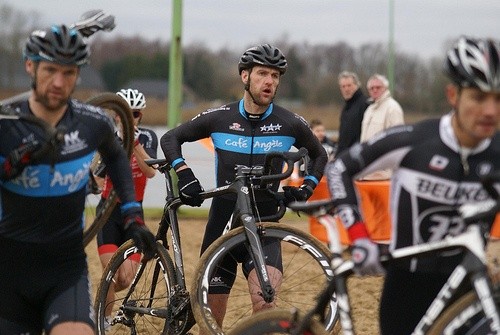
[305,175,318,185]
[172,158,186,172]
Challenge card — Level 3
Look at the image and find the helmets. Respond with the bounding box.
[447,36,500,94]
[115,88,146,109]
[238,44,287,76]
[23,25,88,65]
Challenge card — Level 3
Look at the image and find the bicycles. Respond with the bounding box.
[230,195,500,334]
[92,159,340,335]
[0,9,136,251]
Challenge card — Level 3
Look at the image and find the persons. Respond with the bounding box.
[160,43,328,335]
[0,24,158,335]
[94,88,159,331]
[333,71,370,155]
[361,73,405,142]
[310,120,336,154]
[327,35,500,335]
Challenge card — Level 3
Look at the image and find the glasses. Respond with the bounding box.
[132,112,140,118]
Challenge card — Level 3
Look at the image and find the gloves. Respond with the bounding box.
[351,237,386,278]
[176,167,205,207]
[122,213,156,263]
[282,180,316,206]
[0,140,42,182]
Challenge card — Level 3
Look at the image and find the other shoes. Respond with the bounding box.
[103,317,111,332]
